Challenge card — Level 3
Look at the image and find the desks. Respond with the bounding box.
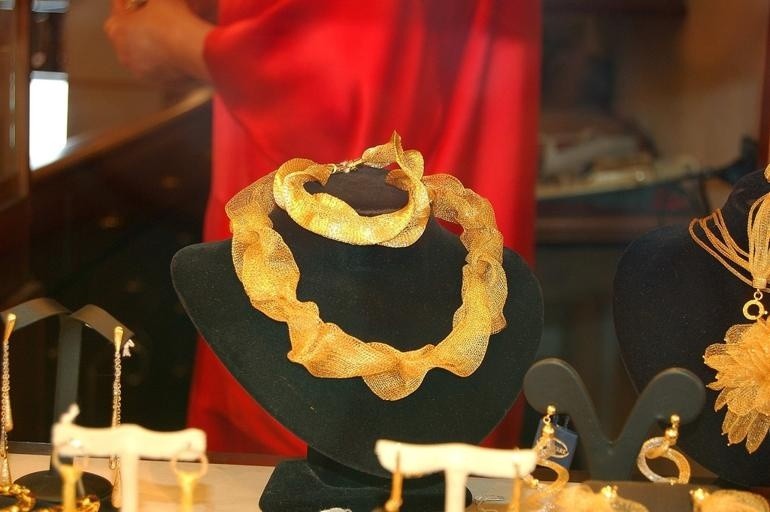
[0,442,770,512]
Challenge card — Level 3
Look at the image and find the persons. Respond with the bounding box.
[106,3,543,465]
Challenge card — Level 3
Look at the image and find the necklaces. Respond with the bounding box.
[702,165,770,455]
[106,326,135,509]
[224,129,508,402]
[1,312,16,497]
[688,209,770,294]
